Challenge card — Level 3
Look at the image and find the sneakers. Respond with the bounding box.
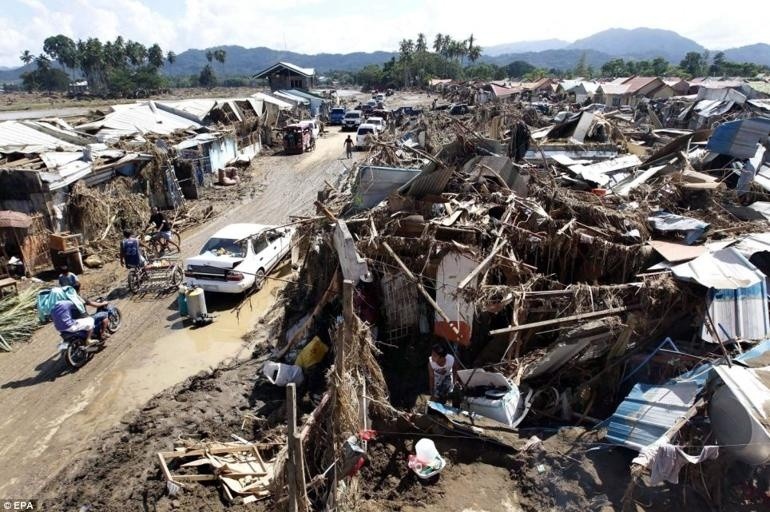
[86,333,112,348]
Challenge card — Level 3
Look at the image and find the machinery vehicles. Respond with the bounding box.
[282,117,321,154]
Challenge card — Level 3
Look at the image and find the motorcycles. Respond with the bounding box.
[61,296,123,370]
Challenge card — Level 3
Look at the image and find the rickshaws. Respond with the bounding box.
[128,252,184,293]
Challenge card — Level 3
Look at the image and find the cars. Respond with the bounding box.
[184,222,297,294]
[328,89,394,150]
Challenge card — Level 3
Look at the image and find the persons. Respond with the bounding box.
[177,284,198,316]
[48,287,100,346]
[59,265,81,295]
[428,343,463,409]
[343,135,355,159]
[142,205,181,253]
[71,281,111,339]
[120,228,153,278]
[735,158,756,206]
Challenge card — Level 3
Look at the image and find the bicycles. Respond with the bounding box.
[139,228,183,256]
